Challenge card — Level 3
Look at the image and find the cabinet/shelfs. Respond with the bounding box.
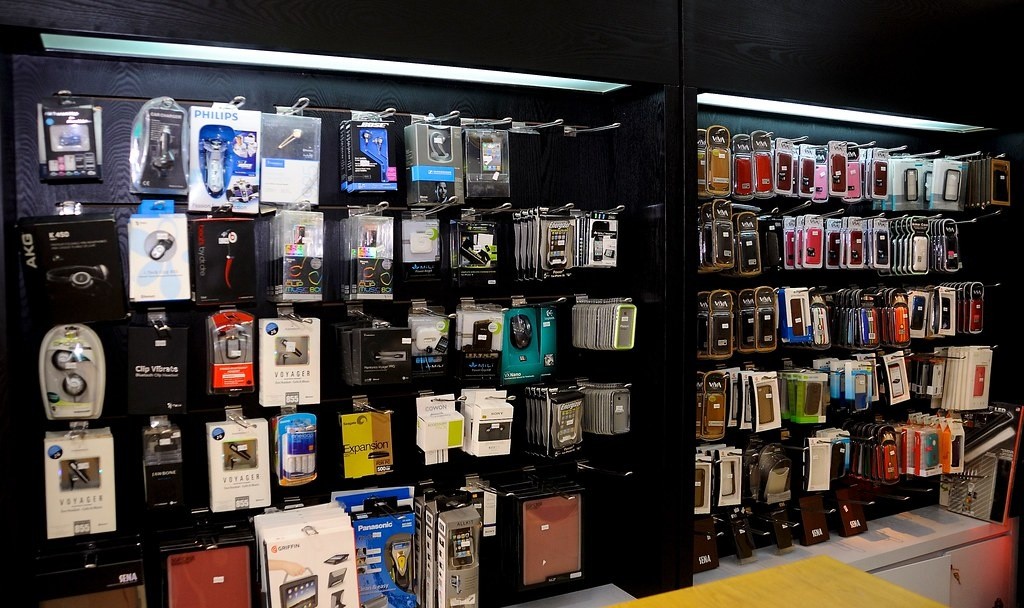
[694,503,1020,608]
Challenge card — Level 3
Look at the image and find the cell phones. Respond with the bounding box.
[604,249,614,258]
[480,135,503,175]
[593,235,604,261]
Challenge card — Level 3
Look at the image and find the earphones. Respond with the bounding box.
[375,138,383,151]
[363,132,370,146]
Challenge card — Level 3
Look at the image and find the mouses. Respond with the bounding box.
[510,314,533,351]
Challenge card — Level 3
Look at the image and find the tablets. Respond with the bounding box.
[280,574,319,608]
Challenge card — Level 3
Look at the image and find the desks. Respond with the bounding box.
[601,553,952,608]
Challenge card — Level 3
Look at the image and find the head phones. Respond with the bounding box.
[47,264,110,289]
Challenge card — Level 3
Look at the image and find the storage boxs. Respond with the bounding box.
[128,212,192,302]
[834,487,869,538]
[794,496,830,548]
[328,321,412,387]
[339,119,464,205]
[42,430,116,539]
[185,106,262,215]
[349,487,480,608]
[43,106,99,179]
[127,327,188,415]
[692,516,719,574]
[205,418,271,513]
[340,411,393,480]
[257,316,321,407]
[191,217,258,305]
[416,388,514,457]
[14,213,128,326]
[259,113,322,204]
